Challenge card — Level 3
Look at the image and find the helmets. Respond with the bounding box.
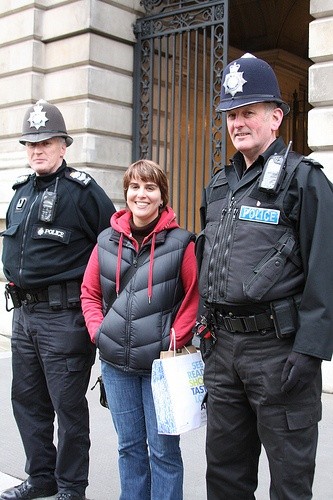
[19,103,74,147]
[215,52,290,116]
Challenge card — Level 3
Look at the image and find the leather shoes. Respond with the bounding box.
[0,479,58,500]
[55,492,85,500]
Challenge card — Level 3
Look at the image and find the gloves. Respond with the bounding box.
[281,351,322,394]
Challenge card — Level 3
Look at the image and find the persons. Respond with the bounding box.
[197,53,332,500]
[0,99,116,500]
[78,159,199,500]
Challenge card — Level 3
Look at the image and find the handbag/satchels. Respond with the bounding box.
[151,327,208,436]
[98,376,110,409]
